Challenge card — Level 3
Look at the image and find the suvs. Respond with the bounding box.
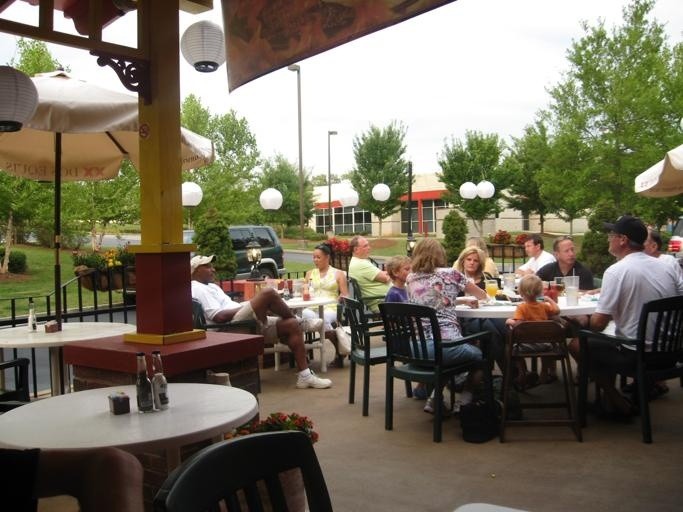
[123,223,282,306]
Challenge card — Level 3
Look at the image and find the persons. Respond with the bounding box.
[189,236,392,389]
[0,402,145,511]
[384,211,681,439]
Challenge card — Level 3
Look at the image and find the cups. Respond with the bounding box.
[502,272,563,304]
[564,276,580,306]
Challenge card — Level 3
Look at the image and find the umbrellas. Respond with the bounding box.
[0,63,216,395]
[634,140,682,201]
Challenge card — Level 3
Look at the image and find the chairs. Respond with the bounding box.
[0,357,30,412]
[155,430,333,512]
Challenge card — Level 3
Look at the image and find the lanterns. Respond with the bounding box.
[0,66,39,133]
[371,183,391,202]
[181,181,203,208]
[476,180,495,199]
[459,181,477,200]
[180,20,228,73]
[259,187,284,211]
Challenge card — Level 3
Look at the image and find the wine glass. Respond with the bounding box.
[486,279,498,306]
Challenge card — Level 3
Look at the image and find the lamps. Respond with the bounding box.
[244,232,266,281]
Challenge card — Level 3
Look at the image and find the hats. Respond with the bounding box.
[600,215,647,244]
[191,255,216,273]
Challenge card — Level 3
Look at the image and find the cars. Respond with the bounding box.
[668,216,683,267]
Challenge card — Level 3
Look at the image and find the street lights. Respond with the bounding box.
[328,130,337,237]
[286,65,304,244]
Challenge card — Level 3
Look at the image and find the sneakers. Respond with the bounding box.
[301,319,322,330]
[297,370,331,388]
[413,389,450,416]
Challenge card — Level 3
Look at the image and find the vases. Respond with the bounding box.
[218,465,306,512]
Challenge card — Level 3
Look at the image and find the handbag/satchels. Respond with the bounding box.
[336,323,352,355]
[460,377,521,443]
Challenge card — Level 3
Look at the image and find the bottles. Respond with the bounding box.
[276,272,313,303]
[135,352,152,412]
[152,351,169,409]
[27,301,36,332]
